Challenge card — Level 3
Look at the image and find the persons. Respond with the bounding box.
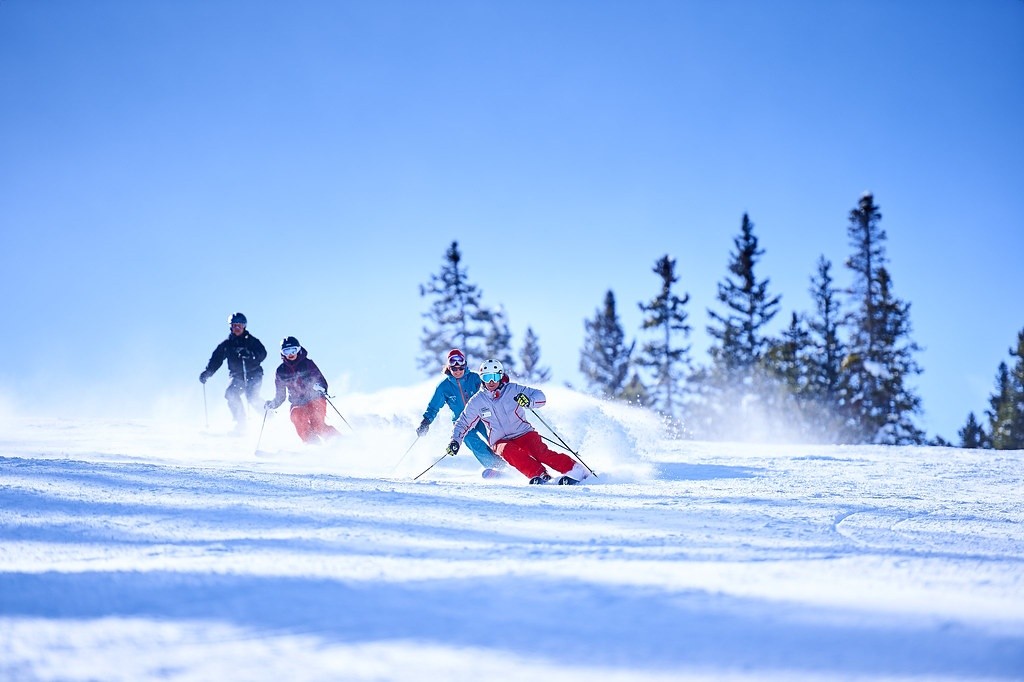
[416,348,590,486]
[265,336,343,451]
[199,313,272,432]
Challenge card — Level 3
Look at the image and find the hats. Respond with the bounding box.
[448,351,465,368]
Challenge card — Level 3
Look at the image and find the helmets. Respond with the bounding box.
[479,359,504,381]
[228,312,246,324]
[281,337,299,348]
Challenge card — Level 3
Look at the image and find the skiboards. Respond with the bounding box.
[481,469,509,479]
[527,472,583,486]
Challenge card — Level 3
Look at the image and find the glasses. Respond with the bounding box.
[480,373,502,383]
[449,354,466,366]
[231,324,244,331]
[281,346,298,356]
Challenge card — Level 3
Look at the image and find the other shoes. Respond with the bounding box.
[540,472,551,481]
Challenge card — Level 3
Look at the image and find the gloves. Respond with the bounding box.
[416,420,429,437]
[264,400,275,409]
[514,393,529,406]
[447,440,459,456]
[237,347,251,360]
[200,371,210,384]
